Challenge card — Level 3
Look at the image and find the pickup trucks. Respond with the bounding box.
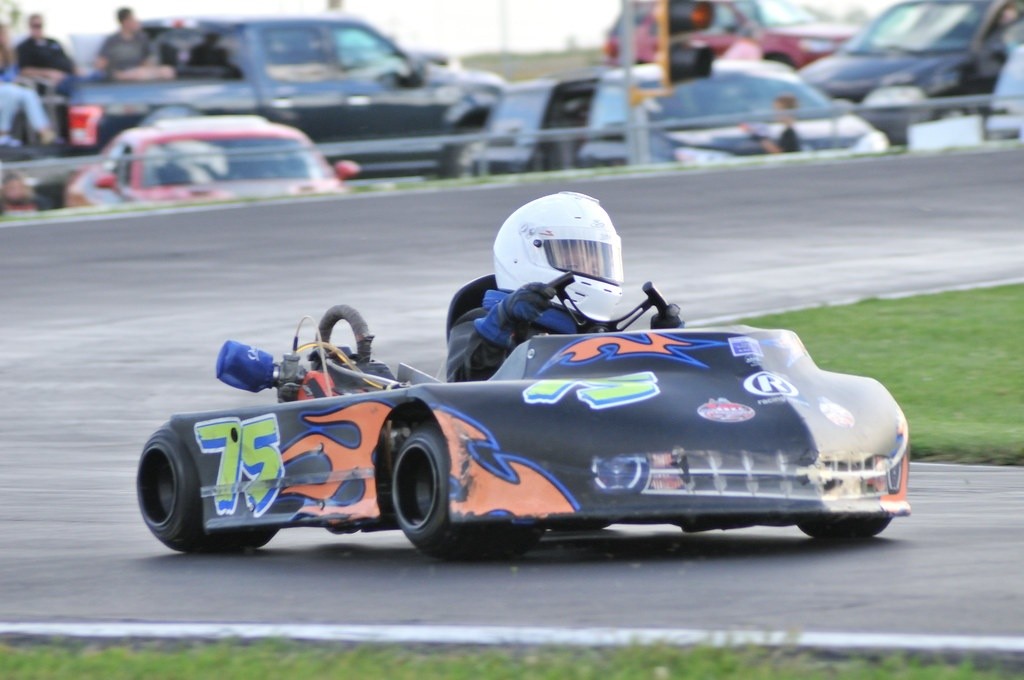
[1,12,506,179]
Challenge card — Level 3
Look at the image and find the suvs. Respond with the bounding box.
[602,0,859,76]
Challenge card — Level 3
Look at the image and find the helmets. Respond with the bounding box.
[493,191,625,322]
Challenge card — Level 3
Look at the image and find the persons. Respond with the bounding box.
[164,29,242,81]
[443,188,686,387]
[742,94,801,155]
[0,171,58,218]
[10,6,163,83]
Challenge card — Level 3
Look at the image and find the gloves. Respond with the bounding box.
[503,281,557,324]
[651,304,686,329]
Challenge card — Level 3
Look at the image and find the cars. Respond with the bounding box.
[795,0,1006,100]
[633,62,892,167]
[442,67,666,179]
[992,46,1024,141]
[63,115,360,209]
